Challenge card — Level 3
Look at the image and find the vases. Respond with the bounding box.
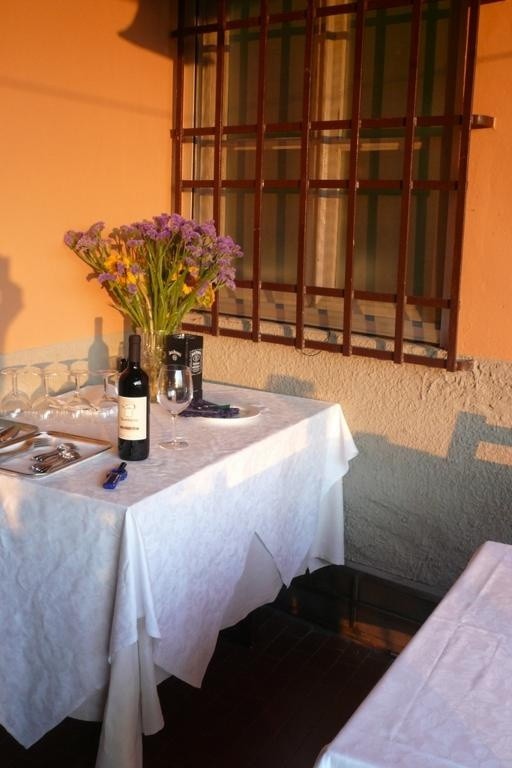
[141,330,166,403]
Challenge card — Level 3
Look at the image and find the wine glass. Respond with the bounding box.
[156,364,193,451]
[0,367,119,428]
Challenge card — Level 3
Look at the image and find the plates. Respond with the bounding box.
[197,401,262,423]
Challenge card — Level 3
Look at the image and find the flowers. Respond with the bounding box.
[63,213,244,402]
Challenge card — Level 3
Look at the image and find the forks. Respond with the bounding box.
[0,437,81,475]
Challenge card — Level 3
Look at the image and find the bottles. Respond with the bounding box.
[118,334,150,461]
